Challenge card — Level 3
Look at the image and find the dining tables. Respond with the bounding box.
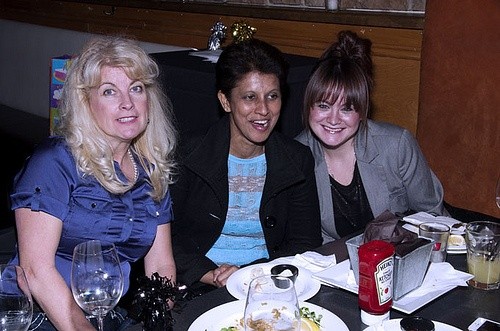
[112,225,500,331]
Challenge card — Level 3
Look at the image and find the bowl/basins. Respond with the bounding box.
[344,233,436,301]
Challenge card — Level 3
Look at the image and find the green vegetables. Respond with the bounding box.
[299,307,323,329]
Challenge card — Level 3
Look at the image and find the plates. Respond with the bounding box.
[188,300,350,331]
[225,262,321,302]
[311,258,475,314]
[363,317,463,331]
[446,250,467,254]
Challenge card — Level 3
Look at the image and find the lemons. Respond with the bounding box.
[300,318,319,331]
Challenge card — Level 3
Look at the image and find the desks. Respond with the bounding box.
[147,47,324,140]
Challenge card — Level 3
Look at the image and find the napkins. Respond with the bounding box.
[268,250,337,288]
[403,211,474,235]
[347,262,468,298]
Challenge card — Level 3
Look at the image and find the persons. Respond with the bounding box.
[0,34,182,331]
[292,30,453,249]
[170,39,322,300]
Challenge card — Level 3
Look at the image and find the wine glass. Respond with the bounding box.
[70,240,125,331]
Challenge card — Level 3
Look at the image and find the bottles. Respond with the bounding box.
[358,240,394,325]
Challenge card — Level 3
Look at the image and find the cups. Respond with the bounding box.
[0,264,34,331]
[244,275,301,331]
[465,220,500,290]
[400,315,435,331]
[418,221,450,264]
[270,264,299,289]
